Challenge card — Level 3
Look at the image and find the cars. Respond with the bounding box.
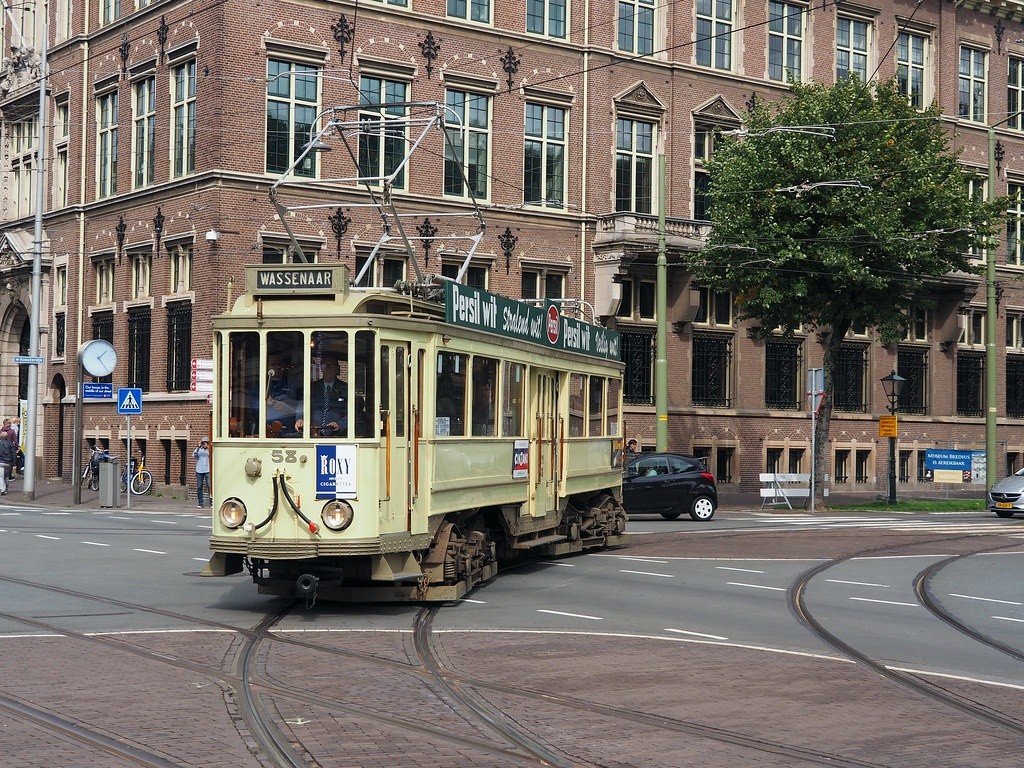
[987,466,1024,519]
[622,454,718,521]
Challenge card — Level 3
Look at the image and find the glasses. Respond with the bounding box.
[321,363,337,368]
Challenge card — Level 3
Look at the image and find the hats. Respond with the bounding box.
[201,437,209,441]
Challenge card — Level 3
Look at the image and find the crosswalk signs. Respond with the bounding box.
[118,388,142,413]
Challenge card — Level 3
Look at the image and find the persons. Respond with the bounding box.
[192,437,213,508]
[292,353,348,438]
[432,377,494,438]
[641,460,657,476]
[250,355,298,437]
[625,440,637,462]
[0,419,25,495]
[354,393,374,437]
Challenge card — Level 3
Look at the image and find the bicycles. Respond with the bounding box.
[79,446,115,490]
[119,450,152,495]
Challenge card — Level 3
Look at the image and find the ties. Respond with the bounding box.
[321,385,330,434]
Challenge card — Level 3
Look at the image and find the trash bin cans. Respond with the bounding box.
[98,453,121,509]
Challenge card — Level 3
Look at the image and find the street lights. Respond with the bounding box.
[881,370,907,506]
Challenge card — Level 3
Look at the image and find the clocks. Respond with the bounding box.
[81,340,118,378]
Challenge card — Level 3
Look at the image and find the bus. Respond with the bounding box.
[203,265,628,606]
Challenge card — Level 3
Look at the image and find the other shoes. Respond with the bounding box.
[197,505,201,508]
[1,491,7,495]
[9,475,15,481]
[211,506,212,508]
[20,467,24,471]
